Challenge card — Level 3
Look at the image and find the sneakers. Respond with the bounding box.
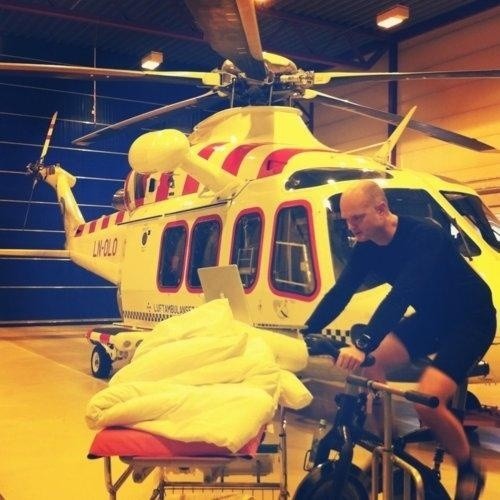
[453,452,487,500]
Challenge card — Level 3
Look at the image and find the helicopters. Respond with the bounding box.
[0,0,500,412]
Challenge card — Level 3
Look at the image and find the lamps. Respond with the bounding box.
[139,52,165,72]
[374,4,409,32]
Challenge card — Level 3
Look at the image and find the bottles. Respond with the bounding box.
[310,417,330,460]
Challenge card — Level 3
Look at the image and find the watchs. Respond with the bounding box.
[355,333,371,350]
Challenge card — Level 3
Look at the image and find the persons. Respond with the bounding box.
[298,176,500,499]
